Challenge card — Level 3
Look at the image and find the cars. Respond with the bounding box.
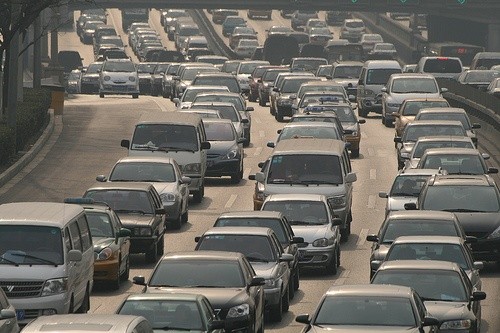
[214,209,305,291]
[256,194,343,274]
[379,167,450,216]
[0,287,21,333]
[193,227,295,323]
[81,182,168,263]
[19,313,154,333]
[367,260,488,333]
[116,292,226,333]
[60,9,500,185]
[370,235,484,301]
[294,285,442,333]
[366,211,477,271]
[56,197,133,293]
[96,157,194,230]
[133,250,266,333]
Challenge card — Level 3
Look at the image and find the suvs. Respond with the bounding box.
[403,172,500,267]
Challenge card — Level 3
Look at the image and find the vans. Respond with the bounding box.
[0,201,95,329]
[248,139,358,242]
[119,111,212,202]
